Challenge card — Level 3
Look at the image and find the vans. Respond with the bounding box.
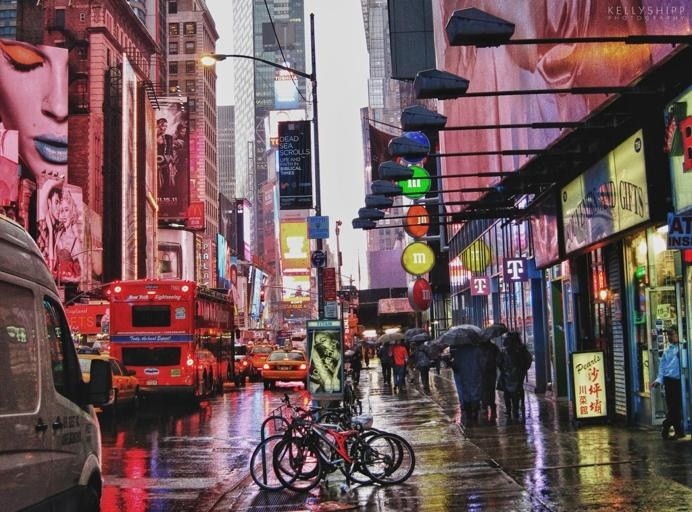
[0,213,114,511]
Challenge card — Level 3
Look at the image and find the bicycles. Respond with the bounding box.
[249,368,417,494]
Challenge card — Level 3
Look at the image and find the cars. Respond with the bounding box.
[69,334,310,422]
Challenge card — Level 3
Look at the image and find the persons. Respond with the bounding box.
[308,331,342,393]
[650,324,684,441]
[39,190,85,284]
[450,345,483,424]
[155,118,176,191]
[34,186,65,271]
[172,120,190,147]
[227,263,238,305]
[0,38,70,181]
[498,332,528,418]
[479,340,501,421]
[356,327,446,397]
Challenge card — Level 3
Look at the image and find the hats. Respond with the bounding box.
[662,324,677,332]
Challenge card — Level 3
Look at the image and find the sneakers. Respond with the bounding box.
[461,415,496,427]
[669,432,685,440]
[662,427,669,439]
[504,406,525,417]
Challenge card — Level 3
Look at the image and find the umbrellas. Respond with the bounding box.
[477,325,507,342]
[439,328,479,348]
[450,323,481,334]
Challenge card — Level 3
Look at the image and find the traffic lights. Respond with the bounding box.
[260,289,264,301]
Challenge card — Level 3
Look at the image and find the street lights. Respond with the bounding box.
[197,11,328,325]
[55,247,106,291]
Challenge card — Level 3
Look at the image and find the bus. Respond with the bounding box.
[107,278,236,401]
